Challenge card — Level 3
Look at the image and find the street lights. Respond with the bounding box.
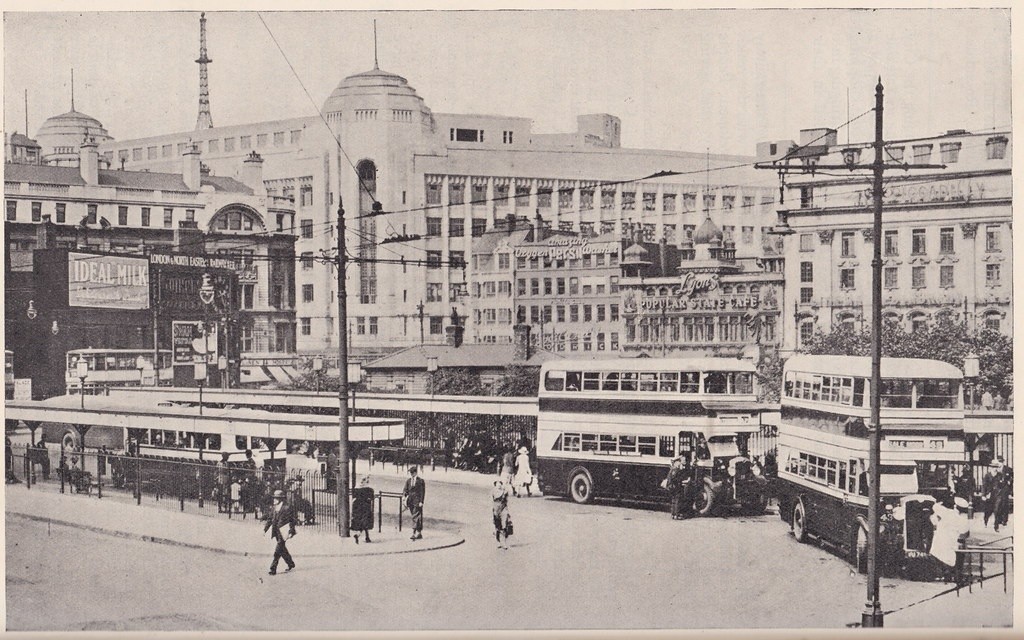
[964,354,979,413]
[136,355,144,390]
[194,360,206,416]
[77,360,87,409]
[754,164,948,628]
[219,356,226,391]
[313,356,323,394]
[347,361,361,421]
[427,357,438,398]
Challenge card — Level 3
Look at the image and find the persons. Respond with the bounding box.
[215,448,313,523]
[5,433,124,492]
[402,467,425,540]
[931,455,1014,584]
[667,456,691,519]
[354,477,380,543]
[750,453,776,507]
[264,490,296,575]
[492,480,513,549]
[501,446,532,497]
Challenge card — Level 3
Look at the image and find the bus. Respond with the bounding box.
[779,355,971,576]
[535,360,770,517]
